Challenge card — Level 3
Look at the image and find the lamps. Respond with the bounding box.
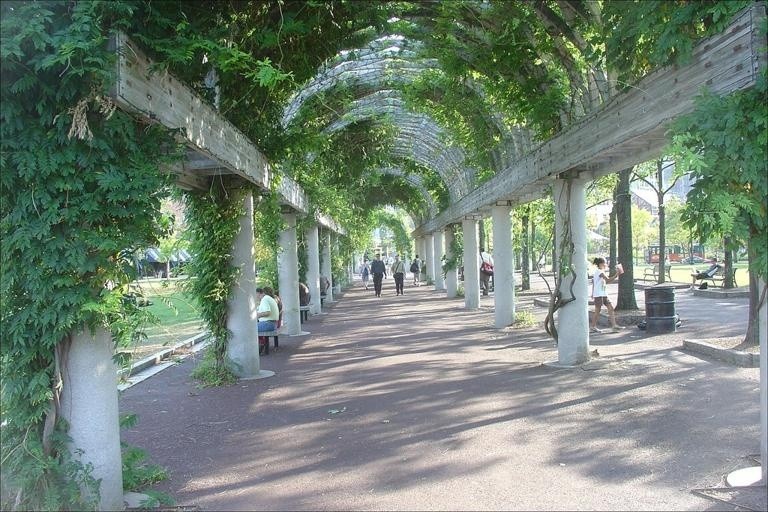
[435,229,441,232]
[464,215,482,220]
[495,200,512,208]
[280,204,293,214]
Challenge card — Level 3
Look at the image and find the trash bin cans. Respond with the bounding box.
[645,287,675,335]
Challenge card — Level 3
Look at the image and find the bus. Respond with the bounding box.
[645,243,705,263]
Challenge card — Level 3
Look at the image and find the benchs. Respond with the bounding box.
[643,263,672,283]
[700,269,738,289]
[320,295,326,309]
[258,326,281,354]
[332,286,337,300]
[300,304,313,324]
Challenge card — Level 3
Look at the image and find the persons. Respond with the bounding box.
[589,257,625,333]
[319,272,330,297]
[256,286,283,355]
[254,288,280,333]
[298,282,311,306]
[358,259,371,290]
[369,254,388,297]
[694,256,722,282]
[369,255,394,277]
[413,255,422,288]
[390,255,407,295]
[479,246,494,296]
